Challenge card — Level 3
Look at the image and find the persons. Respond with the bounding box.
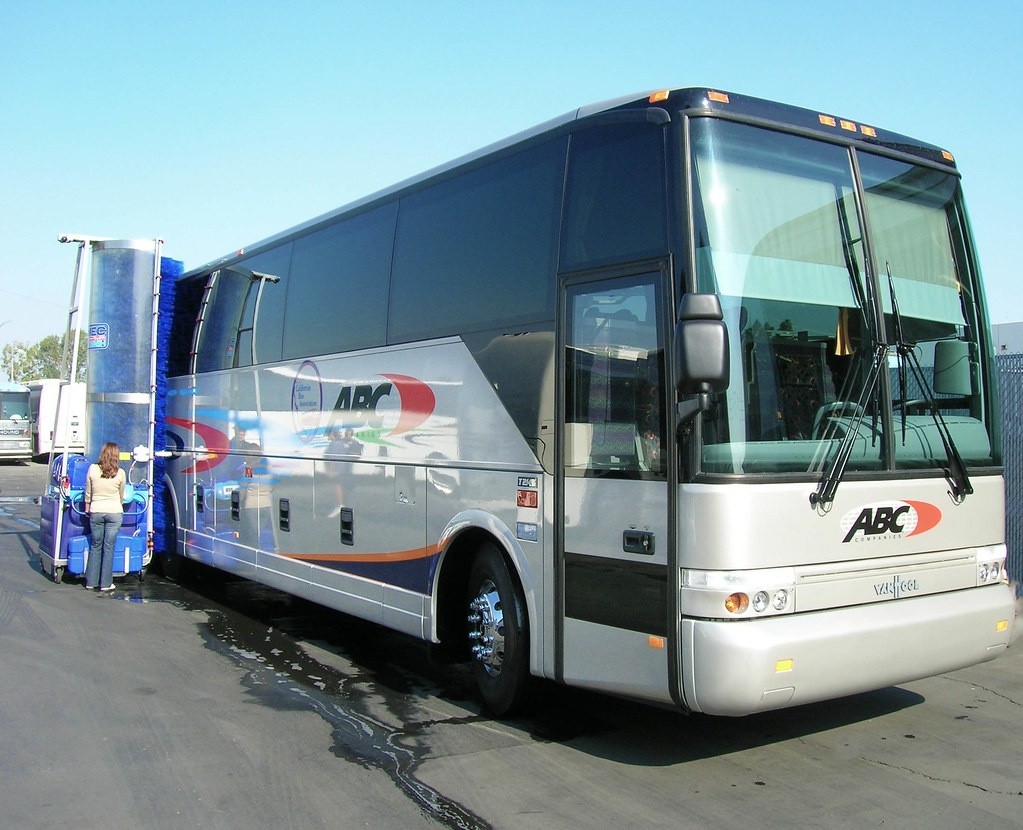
[228,424,277,552]
[323,429,361,519]
[85,443,126,591]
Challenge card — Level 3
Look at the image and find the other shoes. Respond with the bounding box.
[100,583,116,591]
[86,584,99,589]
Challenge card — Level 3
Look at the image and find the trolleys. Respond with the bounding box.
[39,232,164,588]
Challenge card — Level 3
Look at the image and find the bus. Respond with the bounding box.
[0,378,87,465]
[163,86,1015,717]
